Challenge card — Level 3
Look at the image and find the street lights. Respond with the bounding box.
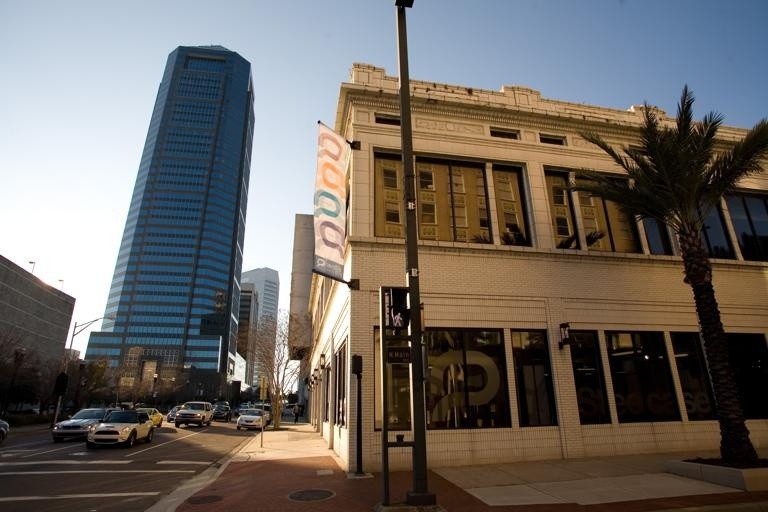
[52,315,116,427]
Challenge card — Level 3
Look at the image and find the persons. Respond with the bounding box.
[293,402,300,423]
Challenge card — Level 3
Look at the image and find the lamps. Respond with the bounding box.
[320,354,330,370]
[558,323,574,348]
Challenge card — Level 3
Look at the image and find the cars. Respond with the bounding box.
[166,401,295,431]
[52,402,163,447]
